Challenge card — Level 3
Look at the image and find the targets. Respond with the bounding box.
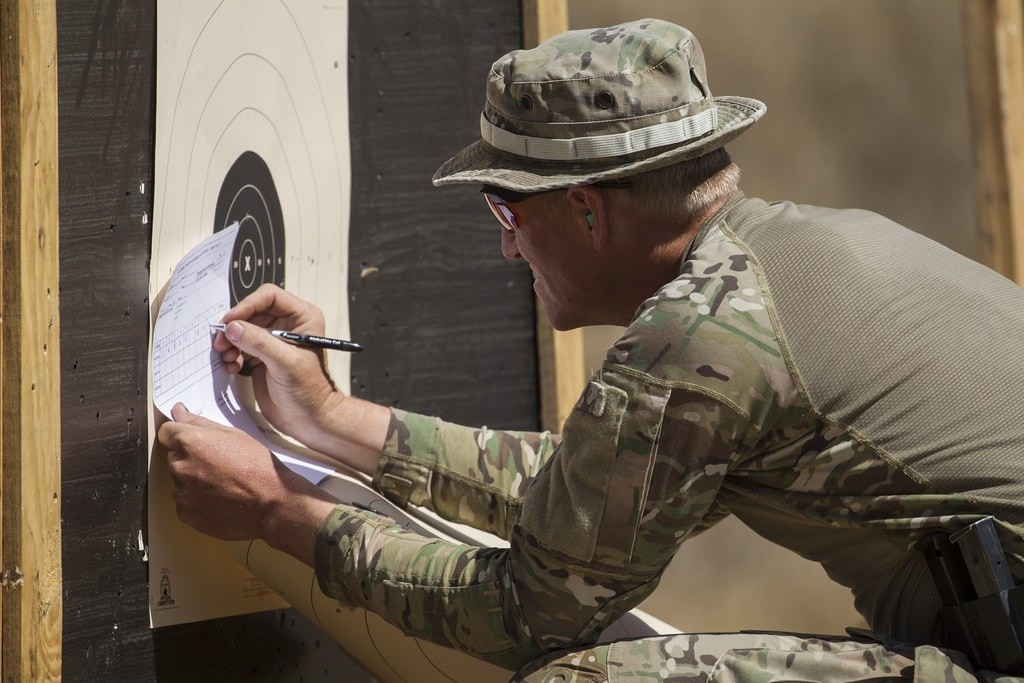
[147,2,349,627]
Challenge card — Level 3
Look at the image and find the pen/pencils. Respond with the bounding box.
[209,323,364,353]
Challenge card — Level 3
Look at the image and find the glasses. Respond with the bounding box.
[479,182,633,233]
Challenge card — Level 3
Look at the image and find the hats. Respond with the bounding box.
[431,18,768,192]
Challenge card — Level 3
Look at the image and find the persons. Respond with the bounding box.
[157,17,1023,683]
